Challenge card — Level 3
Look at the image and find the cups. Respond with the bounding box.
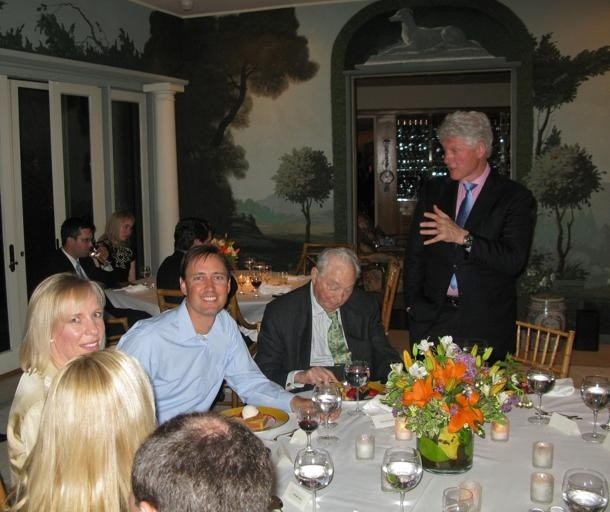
[491,418,508,442]
[443,487,473,512]
[527,438,609,512]
[393,417,413,441]
[355,433,376,461]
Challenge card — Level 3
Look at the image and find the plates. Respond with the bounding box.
[216,405,290,434]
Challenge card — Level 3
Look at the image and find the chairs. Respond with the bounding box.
[508,318,577,381]
[95,215,414,411]
[1,432,14,512]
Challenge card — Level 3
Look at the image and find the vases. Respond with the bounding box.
[407,409,475,475]
[524,290,569,354]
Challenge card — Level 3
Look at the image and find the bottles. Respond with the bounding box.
[396,118,446,199]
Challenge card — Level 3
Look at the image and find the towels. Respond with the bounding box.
[539,374,576,400]
[358,392,394,428]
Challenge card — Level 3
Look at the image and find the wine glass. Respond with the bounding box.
[294,448,334,512]
[295,360,370,449]
[89,247,111,268]
[236,259,289,297]
[523,366,555,428]
[139,267,152,286]
[579,373,609,446]
[382,445,423,511]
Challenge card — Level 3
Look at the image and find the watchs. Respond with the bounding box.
[461,231,473,250]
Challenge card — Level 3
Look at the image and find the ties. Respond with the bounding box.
[327,312,353,365]
[450,183,478,288]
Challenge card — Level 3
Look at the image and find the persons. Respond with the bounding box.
[47,217,155,336]
[10,348,157,512]
[155,217,238,315]
[128,413,275,512]
[114,246,342,425]
[97,210,136,285]
[403,110,537,368]
[6,271,109,479]
[253,247,404,389]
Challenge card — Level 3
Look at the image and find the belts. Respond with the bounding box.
[446,296,462,307]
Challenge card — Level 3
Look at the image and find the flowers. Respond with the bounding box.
[515,245,590,295]
[377,335,532,437]
